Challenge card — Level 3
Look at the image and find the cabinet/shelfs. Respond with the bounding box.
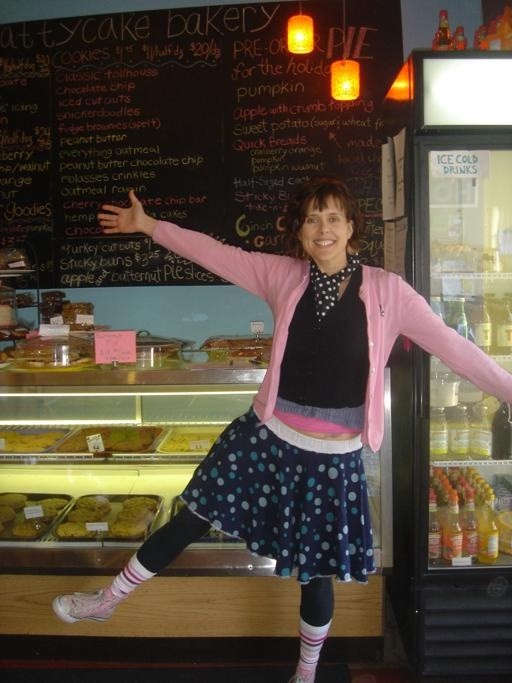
[0,345,384,639]
[0,239,53,363]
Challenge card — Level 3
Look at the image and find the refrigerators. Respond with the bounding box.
[377,123,511,680]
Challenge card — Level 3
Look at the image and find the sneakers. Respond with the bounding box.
[287,664,318,683]
[50,583,123,625]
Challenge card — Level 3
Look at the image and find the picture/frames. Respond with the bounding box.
[413,47,511,137]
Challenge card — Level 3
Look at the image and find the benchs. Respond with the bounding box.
[0,663,353,683]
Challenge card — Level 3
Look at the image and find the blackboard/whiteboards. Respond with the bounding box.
[1,0,405,290]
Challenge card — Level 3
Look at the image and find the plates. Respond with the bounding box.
[1,269,35,275]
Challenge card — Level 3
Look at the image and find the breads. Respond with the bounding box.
[37,498,67,509]
[14,519,44,536]
[0,506,18,522]
[115,508,150,524]
[58,428,163,451]
[16,509,56,520]
[0,494,26,511]
[0,524,4,533]
[56,523,99,537]
[76,496,110,516]
[122,497,157,512]
[67,508,101,523]
[110,519,146,536]
[160,432,217,452]
[0,431,64,452]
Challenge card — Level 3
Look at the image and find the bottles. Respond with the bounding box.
[428,465,500,565]
[430,291,512,459]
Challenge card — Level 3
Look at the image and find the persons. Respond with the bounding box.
[50,176,512,683]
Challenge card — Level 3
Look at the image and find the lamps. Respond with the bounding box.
[330,0,361,102]
[287,0,314,55]
